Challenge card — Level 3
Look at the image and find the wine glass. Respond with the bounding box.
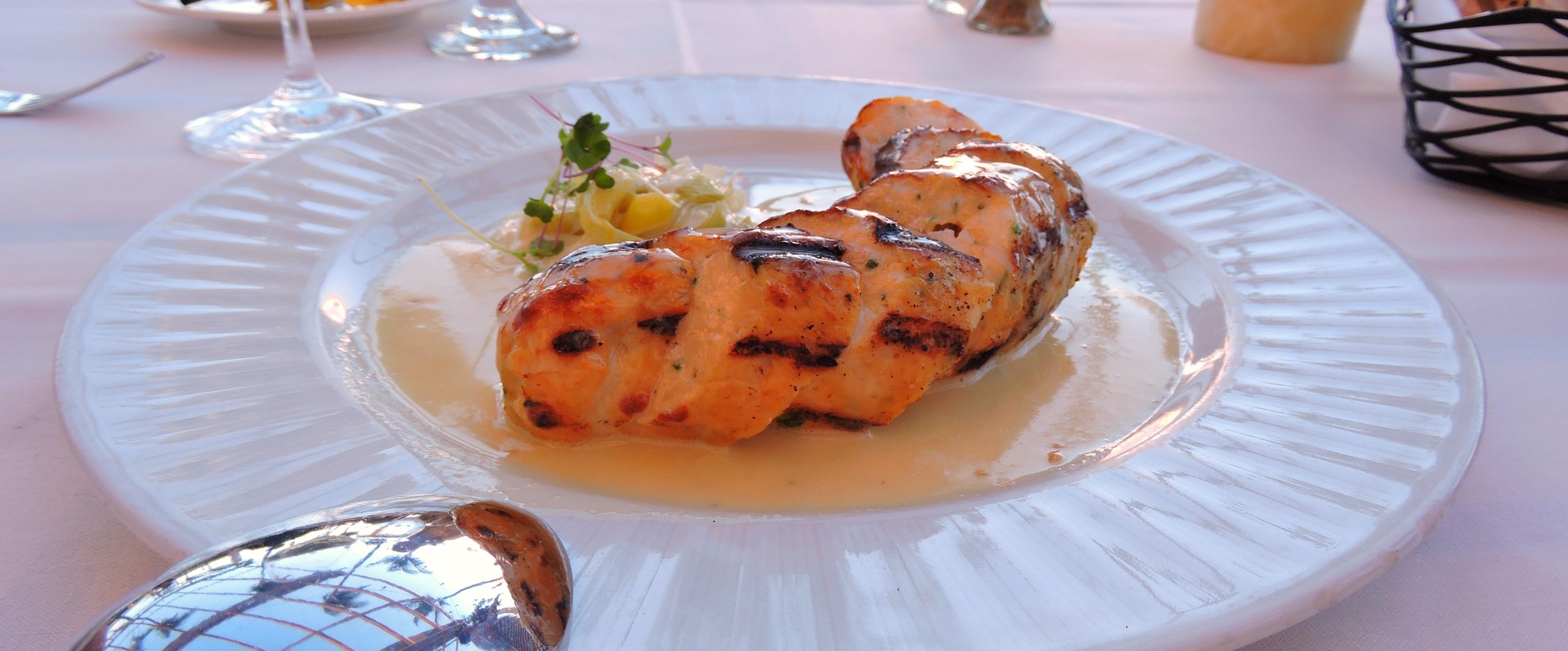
[426,0,578,61]
[181,0,427,161]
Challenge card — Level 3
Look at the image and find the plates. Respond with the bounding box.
[57,72,1486,651]
[130,0,454,34]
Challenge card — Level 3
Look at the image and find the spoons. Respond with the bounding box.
[70,490,573,651]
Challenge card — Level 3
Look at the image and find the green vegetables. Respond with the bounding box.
[416,93,676,269]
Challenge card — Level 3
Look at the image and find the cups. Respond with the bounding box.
[1192,1,1363,65]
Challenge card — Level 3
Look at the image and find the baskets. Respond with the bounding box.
[1386,0,1568,205]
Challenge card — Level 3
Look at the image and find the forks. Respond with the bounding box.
[0,50,165,115]
